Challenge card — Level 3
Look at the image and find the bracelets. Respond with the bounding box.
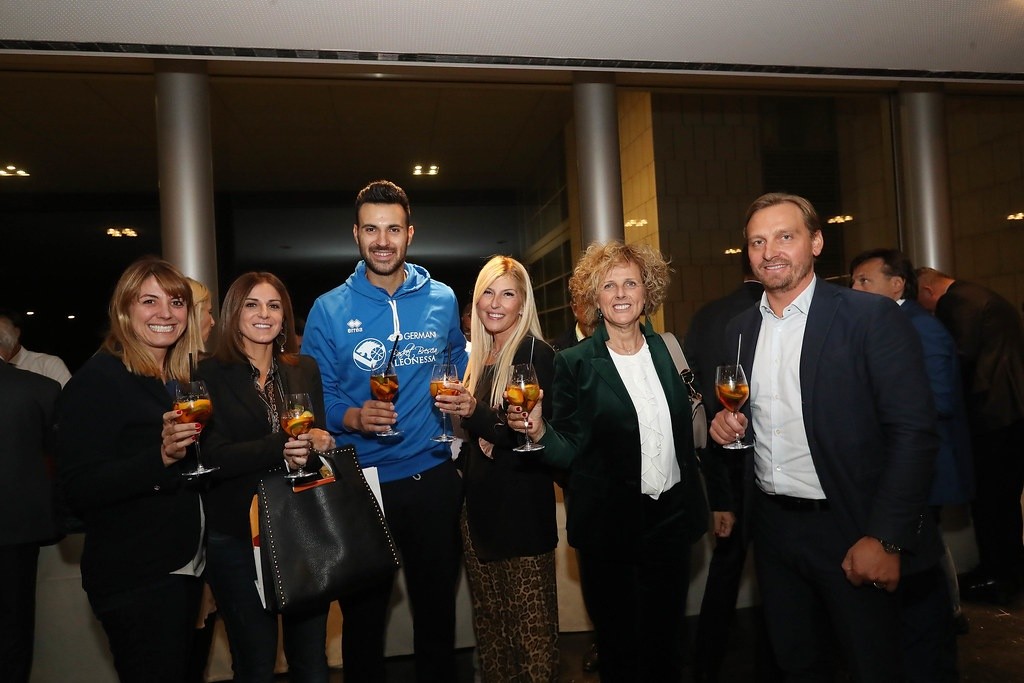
[877,539,902,552]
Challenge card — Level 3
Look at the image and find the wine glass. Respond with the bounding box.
[173,379,220,476]
[371,363,404,436]
[715,364,754,451]
[430,364,460,442]
[281,393,317,478]
[506,363,545,452]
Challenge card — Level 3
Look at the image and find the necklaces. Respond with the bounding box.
[608,339,640,354]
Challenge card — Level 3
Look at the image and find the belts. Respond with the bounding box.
[754,489,827,509]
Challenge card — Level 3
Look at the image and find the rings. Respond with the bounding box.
[873,583,887,592]
[457,406,461,411]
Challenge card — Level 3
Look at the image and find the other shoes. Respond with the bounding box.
[961,582,1007,603]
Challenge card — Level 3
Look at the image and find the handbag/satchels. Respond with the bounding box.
[661,332,707,450]
[259,444,401,613]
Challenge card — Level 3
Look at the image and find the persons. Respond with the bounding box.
[51,260,217,683]
[185,277,215,344]
[300,180,468,683]
[193,272,336,683]
[506,239,737,683]
[708,192,928,683]
[0,317,74,683]
[435,256,558,683]
[917,268,1024,599]
[686,245,783,682]
[850,248,969,683]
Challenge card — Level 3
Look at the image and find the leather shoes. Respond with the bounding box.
[583,640,600,673]
